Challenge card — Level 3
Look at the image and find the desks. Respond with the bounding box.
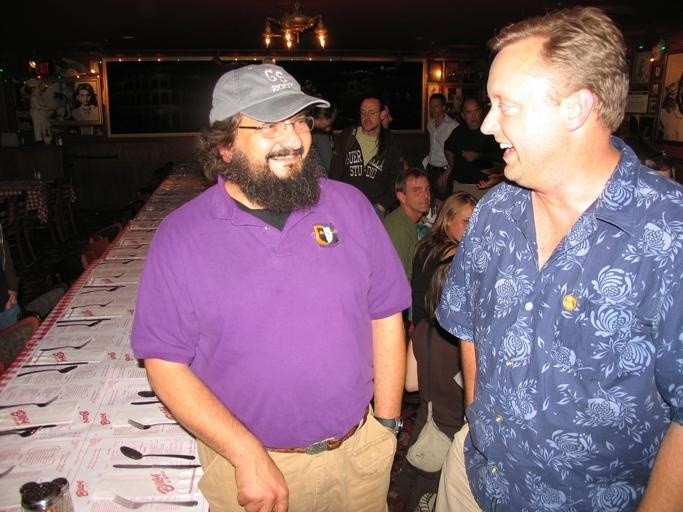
[0,163,241,512]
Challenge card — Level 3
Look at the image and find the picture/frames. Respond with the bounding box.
[620,42,682,149]
[70,75,102,126]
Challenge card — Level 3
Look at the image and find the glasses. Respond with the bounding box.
[236,117,315,138]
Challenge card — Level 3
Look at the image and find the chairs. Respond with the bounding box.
[0,165,164,368]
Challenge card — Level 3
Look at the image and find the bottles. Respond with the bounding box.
[17,477,75,512]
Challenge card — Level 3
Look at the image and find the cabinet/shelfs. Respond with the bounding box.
[423,54,489,117]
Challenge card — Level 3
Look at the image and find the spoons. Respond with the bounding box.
[16,365,77,377]
[118,444,196,461]
[137,390,156,398]
[0,425,41,437]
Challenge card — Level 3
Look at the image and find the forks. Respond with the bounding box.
[127,418,180,432]
[38,244,143,352]
[112,494,199,510]
[0,394,58,410]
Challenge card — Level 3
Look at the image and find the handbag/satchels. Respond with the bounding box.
[405,314,453,474]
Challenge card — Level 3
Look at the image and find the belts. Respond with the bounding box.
[257,403,370,455]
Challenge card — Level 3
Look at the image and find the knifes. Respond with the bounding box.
[113,461,200,469]
[3,423,55,431]
[22,362,90,368]
[129,401,159,405]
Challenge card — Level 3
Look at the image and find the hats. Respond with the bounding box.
[208,63,331,128]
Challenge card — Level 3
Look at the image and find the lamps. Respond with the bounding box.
[259,1,329,53]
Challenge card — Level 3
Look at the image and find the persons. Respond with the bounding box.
[0,226,21,332]
[131,63,413,512]
[433,6,683,512]
[72,83,98,121]
